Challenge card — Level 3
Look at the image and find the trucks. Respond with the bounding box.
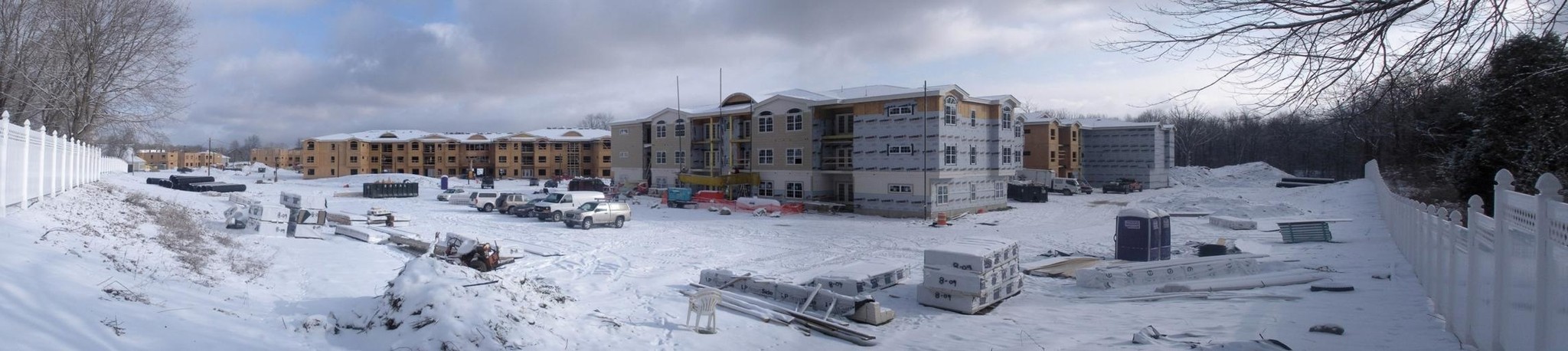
[1014,169,1081,196]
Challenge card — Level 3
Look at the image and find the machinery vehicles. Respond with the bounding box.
[1102,177,1143,194]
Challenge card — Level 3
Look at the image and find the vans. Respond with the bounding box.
[569,179,609,192]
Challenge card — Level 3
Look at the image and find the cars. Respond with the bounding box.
[437,189,469,201]
[1078,180,1093,194]
[529,177,539,186]
[544,180,558,188]
[468,191,631,229]
[481,176,494,188]
